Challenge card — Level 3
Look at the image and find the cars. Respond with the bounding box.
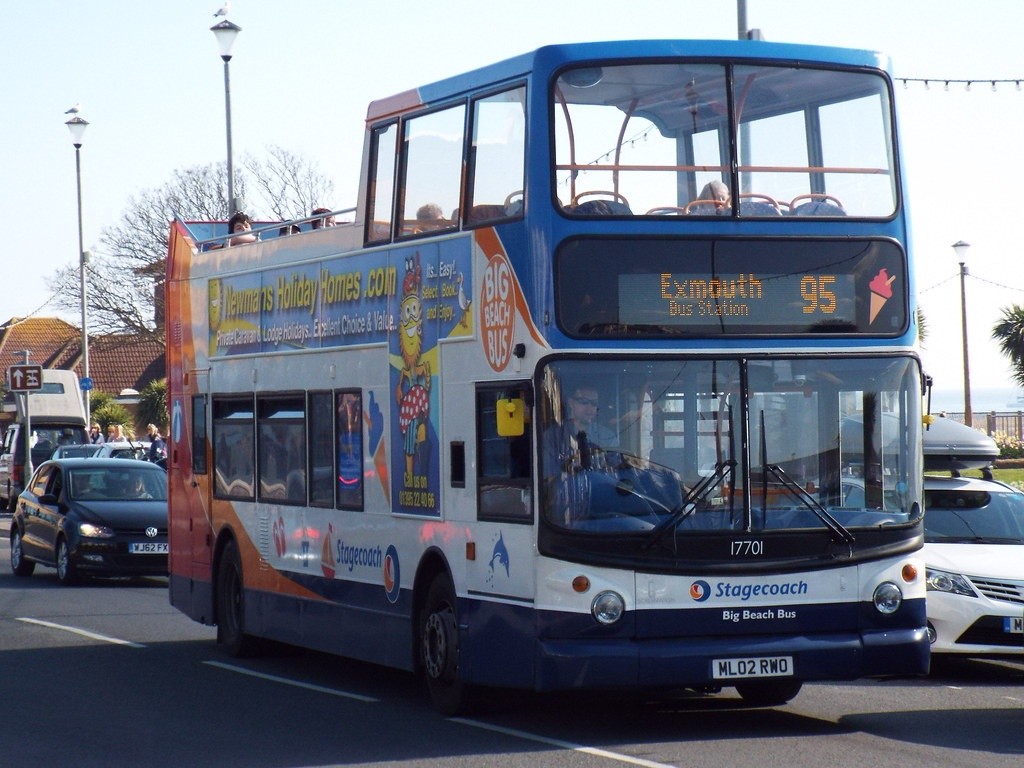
[10,457,169,585]
[800,413,1024,656]
[49,444,100,459]
[90,442,153,460]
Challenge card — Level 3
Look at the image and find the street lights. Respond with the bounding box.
[952,240,973,427]
[209,19,241,221]
[13,350,36,488]
[66,116,91,428]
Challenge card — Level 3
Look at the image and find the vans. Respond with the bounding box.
[0,369,91,500]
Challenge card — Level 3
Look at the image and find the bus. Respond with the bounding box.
[165,41,932,715]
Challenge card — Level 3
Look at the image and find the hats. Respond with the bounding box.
[280,220,300,231]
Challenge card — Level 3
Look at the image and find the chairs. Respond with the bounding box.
[403,180,844,234]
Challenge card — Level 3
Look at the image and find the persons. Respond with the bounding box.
[542,383,619,483]
[147,423,161,442]
[417,204,444,232]
[280,220,301,237]
[123,473,153,497]
[698,181,729,209]
[107,425,126,443]
[228,214,256,246]
[311,208,336,230]
[90,423,104,444]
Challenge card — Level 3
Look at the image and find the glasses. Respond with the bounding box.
[574,397,598,406]
